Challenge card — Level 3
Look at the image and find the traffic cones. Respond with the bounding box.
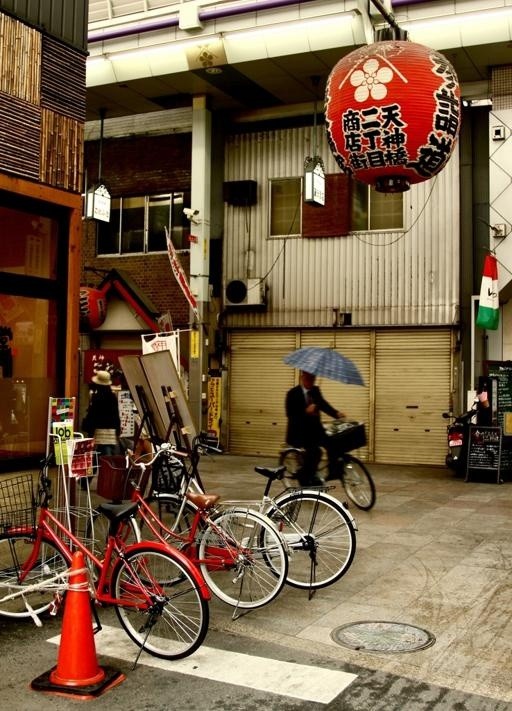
[29,553,128,701]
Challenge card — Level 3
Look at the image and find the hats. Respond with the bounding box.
[91,371,113,386]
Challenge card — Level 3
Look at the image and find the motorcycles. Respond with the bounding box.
[442,395,483,479]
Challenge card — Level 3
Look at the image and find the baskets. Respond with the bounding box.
[97,456,152,500]
[0,474,36,535]
[152,462,185,494]
[330,424,366,452]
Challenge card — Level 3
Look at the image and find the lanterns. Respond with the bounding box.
[323,40,462,192]
[79,285,108,336]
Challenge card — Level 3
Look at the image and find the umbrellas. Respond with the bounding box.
[279,346,366,403]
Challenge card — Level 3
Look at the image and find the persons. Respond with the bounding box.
[285,370,345,487]
[77,370,124,491]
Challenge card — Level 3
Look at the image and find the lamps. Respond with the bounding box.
[303,79,326,207]
[223,278,266,307]
[221,179,257,206]
[83,81,112,223]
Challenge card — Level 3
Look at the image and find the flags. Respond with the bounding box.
[477,255,500,331]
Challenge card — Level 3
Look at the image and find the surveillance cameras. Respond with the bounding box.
[183,208,199,224]
